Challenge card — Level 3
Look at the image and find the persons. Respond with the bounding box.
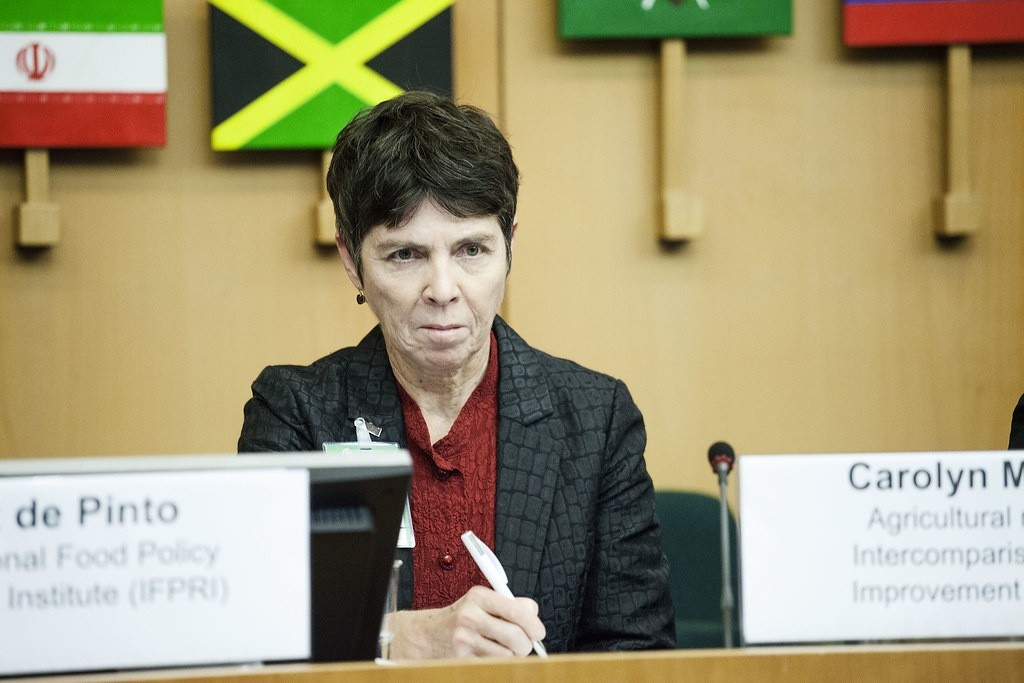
[225,90,682,663]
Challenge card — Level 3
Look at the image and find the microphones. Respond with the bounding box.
[707,442,735,647]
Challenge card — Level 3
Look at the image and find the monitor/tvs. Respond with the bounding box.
[2,450,411,679]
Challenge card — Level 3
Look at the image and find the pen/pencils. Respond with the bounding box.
[460,529,548,658]
